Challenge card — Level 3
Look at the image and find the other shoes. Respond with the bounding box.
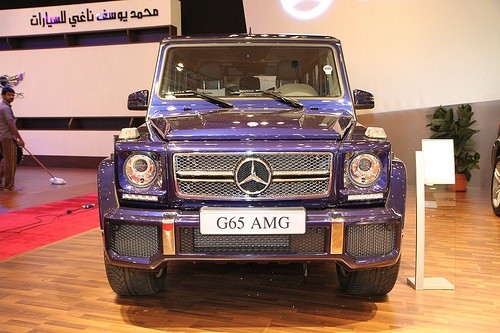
[4,185,23,191]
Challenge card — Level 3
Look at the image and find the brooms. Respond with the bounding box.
[12,136,67,186]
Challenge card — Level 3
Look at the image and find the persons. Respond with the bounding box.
[0,86,25,191]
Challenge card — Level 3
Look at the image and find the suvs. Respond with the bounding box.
[97,27,407,296]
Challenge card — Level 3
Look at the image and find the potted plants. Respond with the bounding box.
[425,103,483,191]
